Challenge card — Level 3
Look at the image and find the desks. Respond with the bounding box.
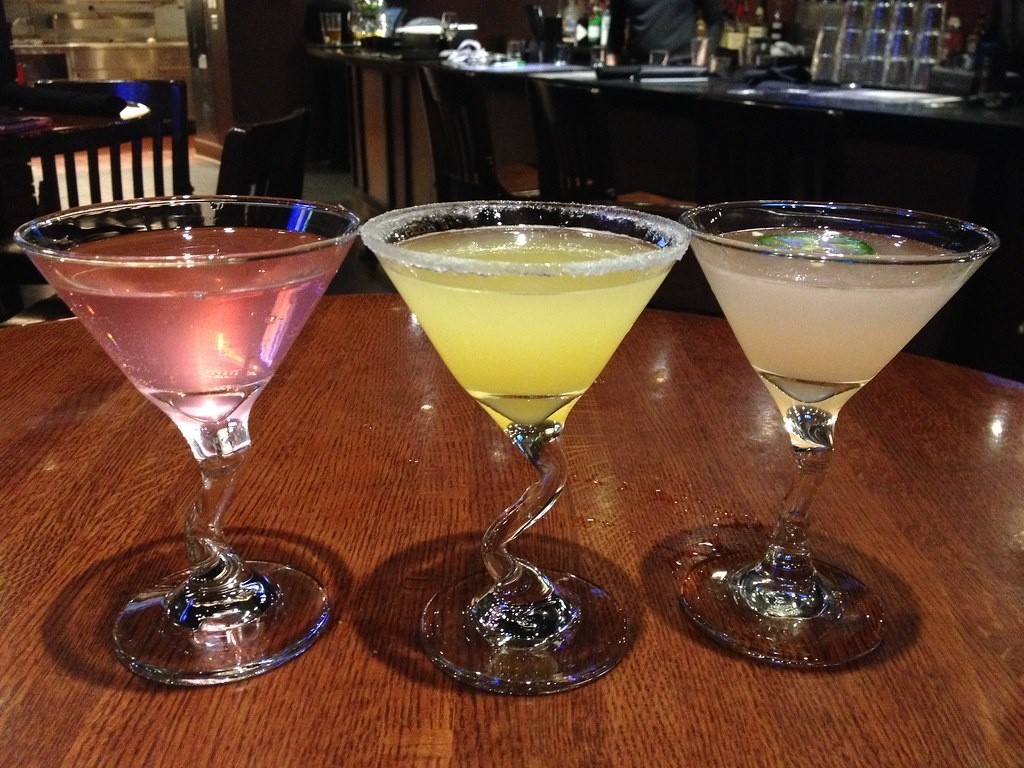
[0,109,155,160]
[0,289,1024,768]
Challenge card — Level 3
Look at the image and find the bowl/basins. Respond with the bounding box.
[395,24,478,54]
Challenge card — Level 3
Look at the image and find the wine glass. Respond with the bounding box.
[680,200,1000,671]
[442,12,458,49]
[14,194,360,686]
[357,199,692,697]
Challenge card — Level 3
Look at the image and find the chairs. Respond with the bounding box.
[418,63,540,201]
[27,77,195,197]
[524,76,701,220]
[213,105,313,198]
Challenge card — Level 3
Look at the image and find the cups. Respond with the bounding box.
[506,40,523,61]
[810,0,948,91]
[591,45,606,70]
[554,42,574,66]
[319,12,342,47]
[650,49,669,67]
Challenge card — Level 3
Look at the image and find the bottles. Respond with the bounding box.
[961,7,988,72]
[561,0,602,47]
[690,3,784,75]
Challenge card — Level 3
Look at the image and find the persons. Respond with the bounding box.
[605,0,723,67]
